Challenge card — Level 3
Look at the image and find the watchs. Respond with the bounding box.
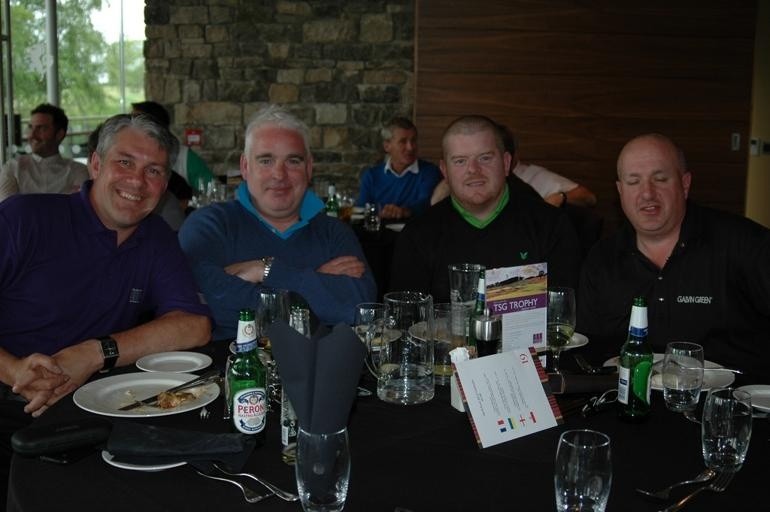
[96,335,120,374]
[260,255,274,282]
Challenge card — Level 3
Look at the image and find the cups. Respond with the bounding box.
[663,342,705,411]
[700,386,754,474]
[313,177,329,205]
[354,303,391,364]
[447,264,482,348]
[338,190,356,226]
[257,287,291,352]
[364,203,381,232]
[428,302,472,386]
[294,426,352,511]
[223,355,270,419]
[364,290,436,406]
[553,428,613,511]
[196,176,233,204]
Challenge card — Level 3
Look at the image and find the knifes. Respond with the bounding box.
[118,366,223,412]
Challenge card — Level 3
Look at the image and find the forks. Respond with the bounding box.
[660,462,737,511]
[663,472,736,511]
[192,468,279,504]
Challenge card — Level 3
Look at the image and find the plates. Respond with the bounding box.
[547,332,590,352]
[350,214,364,224]
[229,339,265,357]
[352,207,365,214]
[411,317,452,344]
[72,372,220,418]
[602,350,736,393]
[135,351,213,373]
[733,384,770,413]
[351,324,402,346]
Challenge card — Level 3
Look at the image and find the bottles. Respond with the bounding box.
[326,183,340,218]
[472,315,502,358]
[617,294,654,421]
[468,269,486,347]
[279,307,312,466]
[227,308,269,437]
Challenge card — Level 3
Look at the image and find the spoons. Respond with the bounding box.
[212,461,311,502]
[635,470,717,500]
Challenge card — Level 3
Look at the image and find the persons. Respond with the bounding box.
[393,114,575,305]
[574,132,770,385]
[429,123,597,210]
[0,113,216,512]
[1,103,222,215]
[178,105,378,343]
[354,117,442,220]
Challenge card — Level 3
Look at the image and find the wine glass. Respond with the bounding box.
[547,286,577,377]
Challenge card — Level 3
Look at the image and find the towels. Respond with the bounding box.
[106,420,257,475]
[268,318,367,502]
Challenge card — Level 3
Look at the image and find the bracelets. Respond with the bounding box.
[558,191,567,208]
